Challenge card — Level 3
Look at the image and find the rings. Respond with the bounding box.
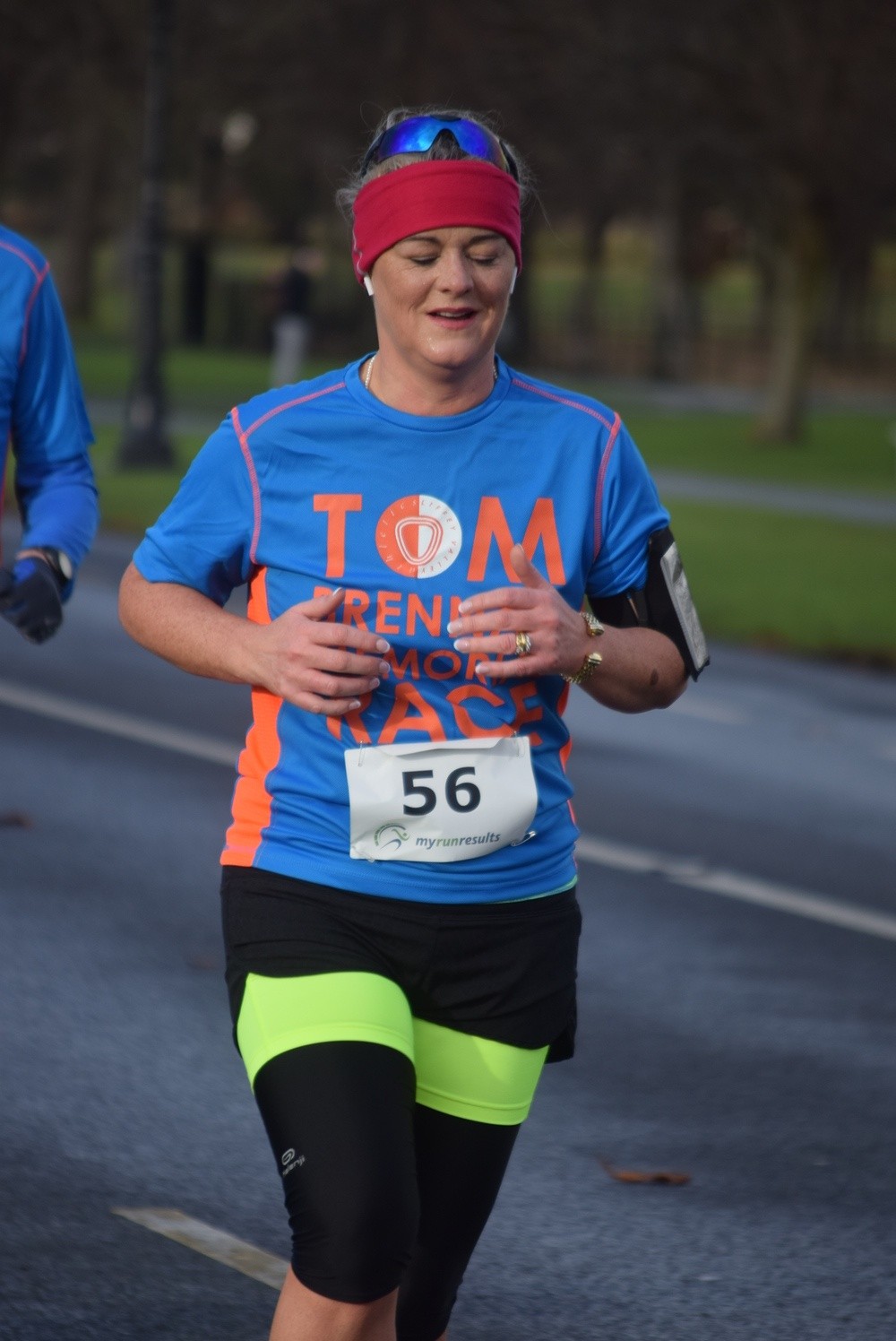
[515,633,532,655]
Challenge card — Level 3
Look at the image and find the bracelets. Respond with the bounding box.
[560,611,605,684]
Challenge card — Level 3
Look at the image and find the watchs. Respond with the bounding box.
[16,545,73,595]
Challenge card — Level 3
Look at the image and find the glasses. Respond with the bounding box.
[360,113,494,175]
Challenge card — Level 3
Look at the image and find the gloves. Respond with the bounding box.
[0,556,63,642]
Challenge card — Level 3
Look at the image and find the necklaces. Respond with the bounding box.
[366,351,498,390]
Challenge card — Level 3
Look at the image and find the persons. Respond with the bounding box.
[0,226,100,646]
[118,107,710,1341]
